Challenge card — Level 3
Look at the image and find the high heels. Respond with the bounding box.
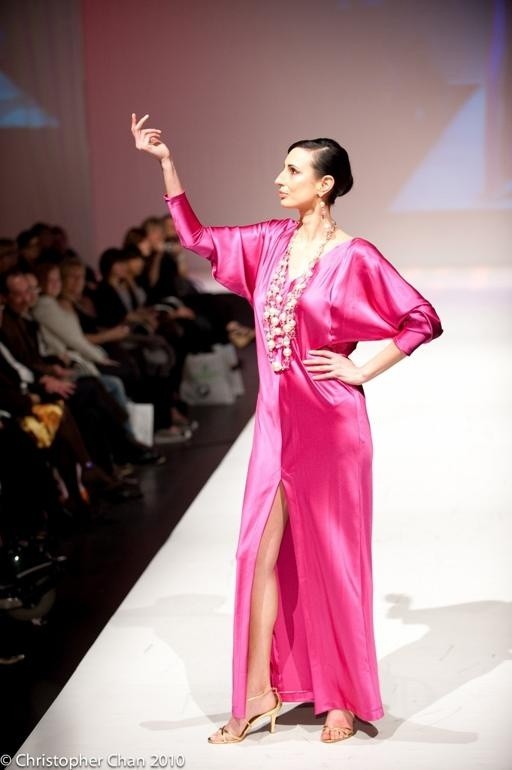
[207,688,281,744]
[320,708,357,743]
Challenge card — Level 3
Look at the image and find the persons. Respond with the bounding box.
[130,109,446,747]
[1,213,256,667]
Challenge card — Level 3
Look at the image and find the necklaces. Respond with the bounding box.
[262,220,337,373]
